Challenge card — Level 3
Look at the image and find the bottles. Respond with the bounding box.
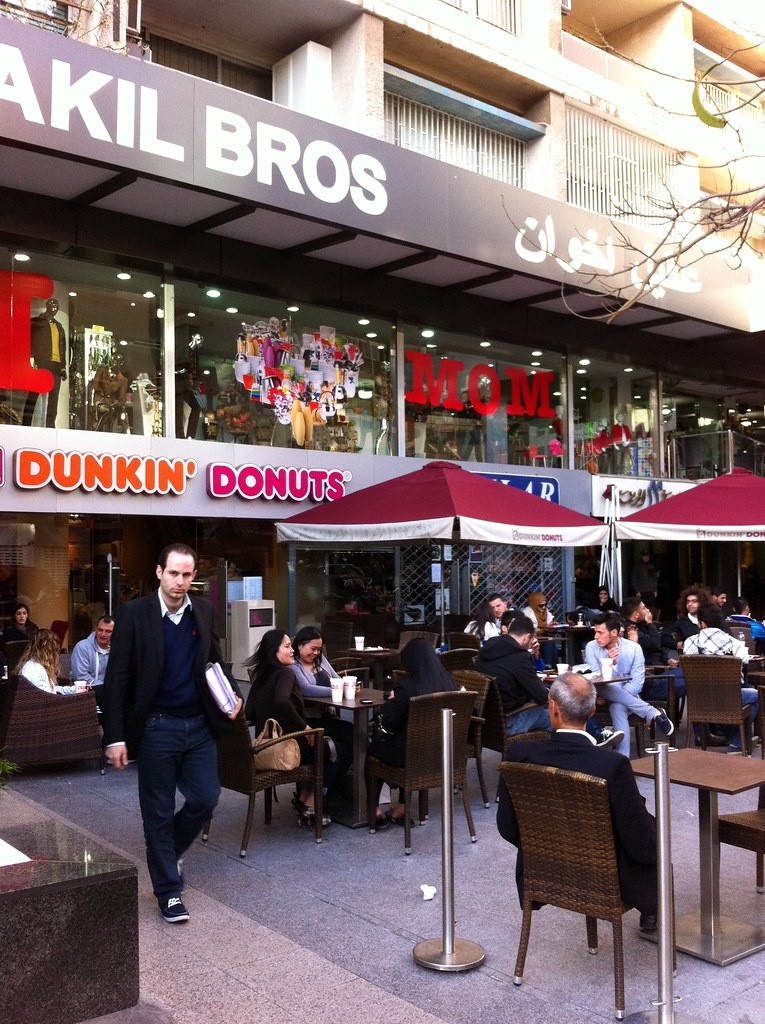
[738,631,746,641]
[578,613,583,626]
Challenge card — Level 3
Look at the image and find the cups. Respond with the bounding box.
[343,676,357,699]
[3,666,8,676]
[600,658,613,679]
[74,681,87,693]
[355,637,365,650]
[585,620,591,627]
[330,678,343,702]
[556,663,569,676]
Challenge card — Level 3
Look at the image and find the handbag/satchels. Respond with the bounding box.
[251,718,301,771]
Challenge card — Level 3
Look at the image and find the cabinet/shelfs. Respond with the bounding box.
[71,328,113,429]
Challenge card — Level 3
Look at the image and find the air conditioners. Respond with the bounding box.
[126,0,143,42]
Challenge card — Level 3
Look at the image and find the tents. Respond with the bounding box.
[613,466,765,596]
[275,461,611,644]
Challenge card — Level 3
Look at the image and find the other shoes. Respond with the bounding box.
[728,744,743,755]
[640,912,657,928]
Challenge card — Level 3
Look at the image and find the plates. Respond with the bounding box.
[2,676,8,679]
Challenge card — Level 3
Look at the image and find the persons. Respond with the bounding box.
[100,544,244,922]
[2,603,39,641]
[11,628,86,695]
[462,405,483,462]
[475,610,625,749]
[682,603,759,755]
[244,629,347,829]
[622,597,687,699]
[464,594,515,648]
[552,406,563,468]
[364,637,456,829]
[92,352,131,434]
[287,626,360,743]
[595,412,632,475]
[631,550,658,606]
[584,612,675,758]
[523,591,558,669]
[21,298,68,428]
[175,332,205,439]
[661,583,765,650]
[589,586,618,612]
[69,615,115,686]
[496,672,658,929]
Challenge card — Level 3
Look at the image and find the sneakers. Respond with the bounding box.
[596,728,624,748]
[158,898,189,922]
[655,707,675,736]
[175,859,185,893]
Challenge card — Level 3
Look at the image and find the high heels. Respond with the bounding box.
[293,798,331,831]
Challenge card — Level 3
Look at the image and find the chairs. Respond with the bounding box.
[216,386,257,445]
[0,619,105,776]
[200,613,765,1022]
[525,446,548,468]
[513,438,529,465]
[548,440,563,468]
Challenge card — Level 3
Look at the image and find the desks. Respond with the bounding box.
[537,624,596,667]
[414,415,486,462]
[337,648,400,722]
[630,747,765,967]
[537,668,632,685]
[301,687,391,828]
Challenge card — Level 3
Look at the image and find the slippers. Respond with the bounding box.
[375,814,387,829]
[385,809,414,826]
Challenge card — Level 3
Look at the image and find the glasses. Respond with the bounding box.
[538,602,548,608]
